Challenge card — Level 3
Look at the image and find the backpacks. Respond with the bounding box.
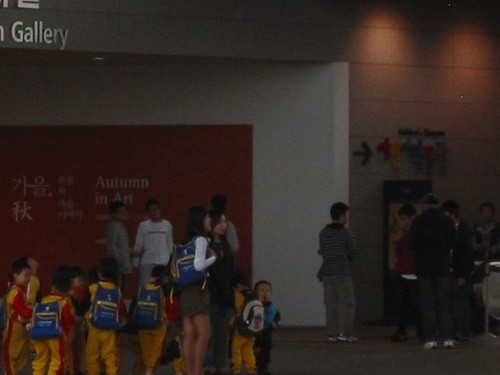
[170,240,205,287]
[26,300,62,339]
[236,295,265,337]
[88,283,122,331]
[130,286,162,330]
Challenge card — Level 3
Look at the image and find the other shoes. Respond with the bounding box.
[424,341,437,350]
[337,334,357,342]
[328,336,336,342]
[443,340,453,349]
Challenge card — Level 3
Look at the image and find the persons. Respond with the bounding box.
[106,201,143,288]
[319,202,357,343]
[227,272,281,375]
[472,202,500,260]
[130,266,174,375]
[131,198,174,283]
[175,193,240,375]
[391,196,475,350]
[0,256,127,375]
